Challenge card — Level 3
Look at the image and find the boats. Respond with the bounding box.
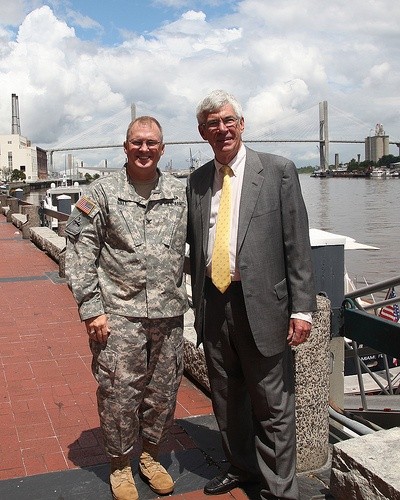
[344,236,400,429]
[39,173,86,232]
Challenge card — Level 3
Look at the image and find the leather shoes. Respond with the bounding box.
[203,470,239,494]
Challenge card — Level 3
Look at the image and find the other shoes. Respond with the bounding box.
[139,452,175,494]
[109,457,139,500]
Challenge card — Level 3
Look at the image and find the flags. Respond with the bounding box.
[379,285,400,366]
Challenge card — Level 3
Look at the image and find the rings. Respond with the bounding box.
[89,331,96,335]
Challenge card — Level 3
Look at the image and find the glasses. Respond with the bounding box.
[200,119,239,128]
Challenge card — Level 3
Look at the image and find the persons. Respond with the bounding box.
[186,89,317,500]
[65,116,189,500]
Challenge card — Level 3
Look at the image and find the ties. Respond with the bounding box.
[211,168,232,293]
